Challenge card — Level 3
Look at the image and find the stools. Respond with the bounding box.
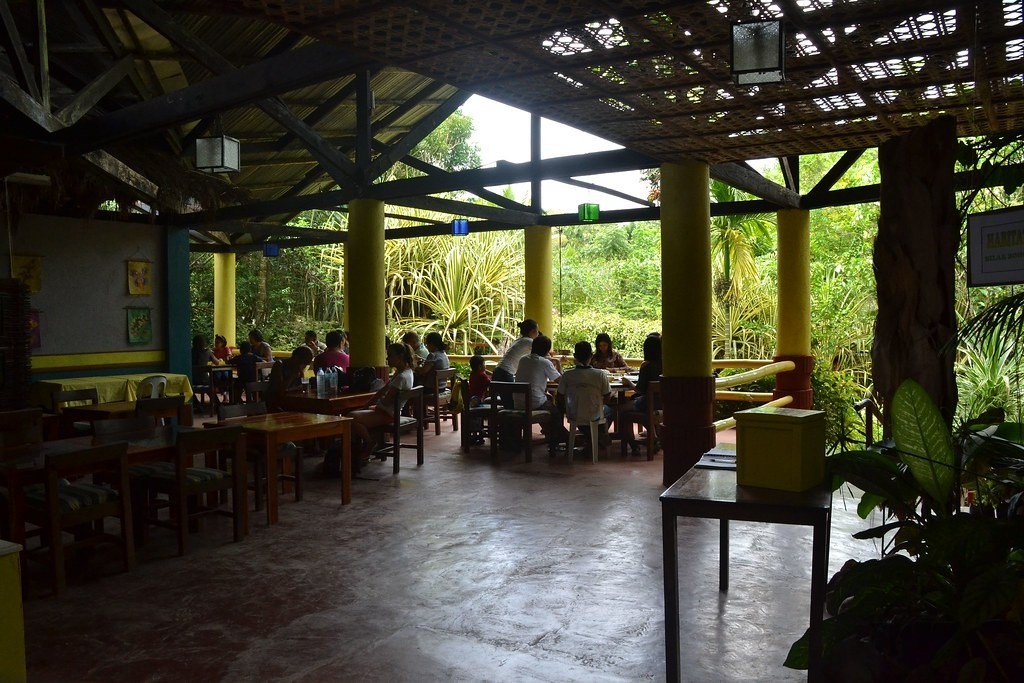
[567,420,612,464]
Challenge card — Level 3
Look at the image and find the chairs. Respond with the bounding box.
[238,362,275,404]
[139,425,246,555]
[364,385,424,474]
[489,381,554,459]
[423,368,458,435]
[621,381,662,461]
[23,441,135,602]
[245,382,267,417]
[460,377,491,449]
[190,366,214,417]
[0,376,184,448]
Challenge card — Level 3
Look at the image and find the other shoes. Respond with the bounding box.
[654,438,661,453]
[632,447,640,456]
[361,440,377,461]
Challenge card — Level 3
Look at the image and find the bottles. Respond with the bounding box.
[331,367,338,386]
[316,367,326,393]
[325,367,332,389]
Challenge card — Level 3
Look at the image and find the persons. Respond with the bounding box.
[347,343,414,465]
[556,341,611,455]
[192,330,271,412]
[614,333,663,455]
[512,331,585,447]
[492,319,539,450]
[385,332,450,433]
[591,334,627,429]
[301,331,327,356]
[314,330,349,390]
[263,346,315,473]
[470,356,491,444]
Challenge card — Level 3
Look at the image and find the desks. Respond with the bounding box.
[202,411,354,526]
[0,425,212,576]
[61,401,137,439]
[659,442,832,683]
[30,372,194,412]
[284,390,379,414]
[546,382,637,388]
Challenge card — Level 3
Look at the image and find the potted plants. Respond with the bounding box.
[782,373,1024,683]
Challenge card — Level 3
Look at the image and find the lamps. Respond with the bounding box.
[578,203,599,222]
[451,216,468,237]
[195,114,241,173]
[264,242,278,257]
[728,17,786,86]
